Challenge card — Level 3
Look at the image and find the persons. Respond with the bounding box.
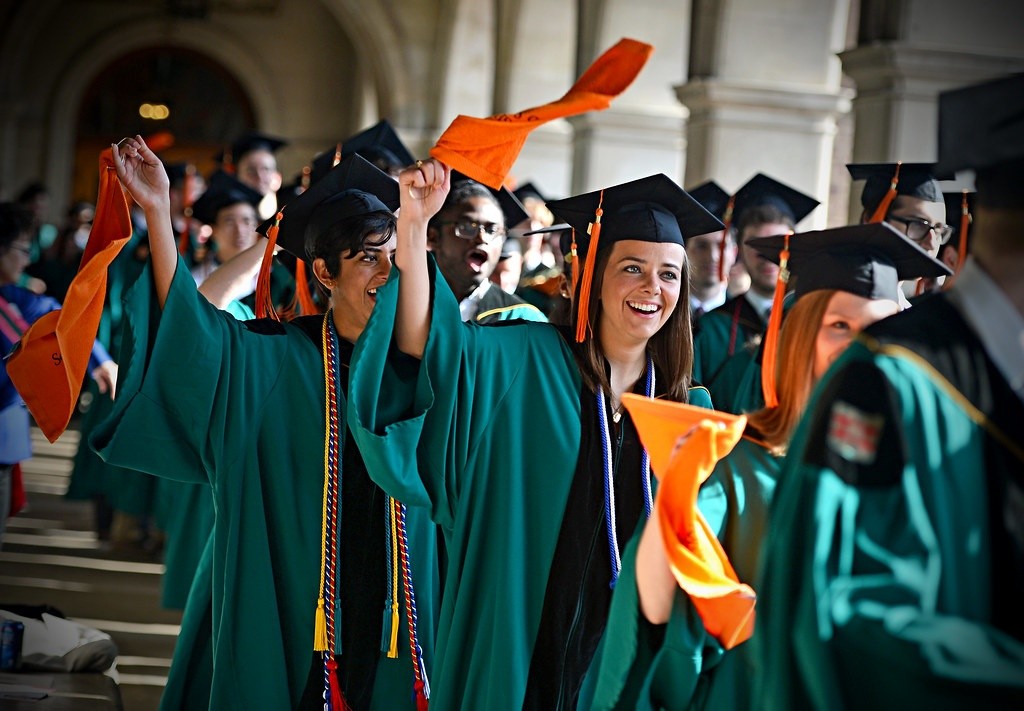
[0,67,1024,711]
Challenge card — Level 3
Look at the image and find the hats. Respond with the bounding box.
[548,173,728,345]
[254,119,420,322]
[745,223,955,407]
[938,71,1024,192]
[938,188,979,289]
[686,179,731,223]
[511,181,545,202]
[524,221,584,304]
[844,159,943,224]
[718,173,821,283]
[178,170,266,257]
[446,167,530,229]
[212,131,290,173]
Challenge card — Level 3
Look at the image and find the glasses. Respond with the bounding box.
[5,243,34,261]
[888,211,955,245]
[436,216,507,243]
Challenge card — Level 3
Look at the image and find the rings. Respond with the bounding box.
[121,156,124,164]
[415,160,422,168]
